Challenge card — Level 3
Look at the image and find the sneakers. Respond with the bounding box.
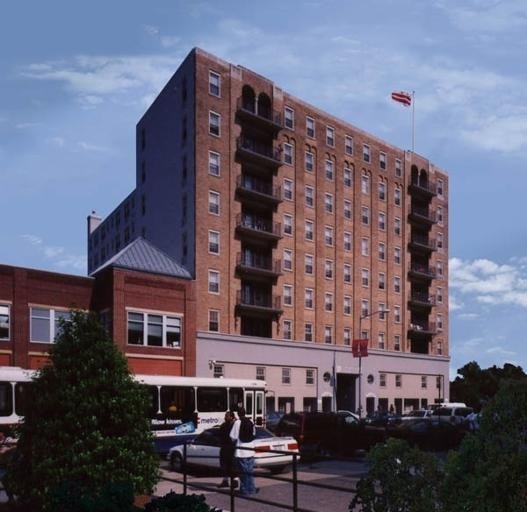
[216,480,240,495]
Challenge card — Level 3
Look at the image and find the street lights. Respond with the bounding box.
[359,309,391,417]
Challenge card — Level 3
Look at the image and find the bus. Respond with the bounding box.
[0,366,279,456]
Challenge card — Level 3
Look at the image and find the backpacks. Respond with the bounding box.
[238,417,255,443]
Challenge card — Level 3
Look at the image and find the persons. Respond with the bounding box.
[165,398,243,412]
[218,412,238,488]
[353,403,394,417]
[229,408,260,496]
[295,410,314,470]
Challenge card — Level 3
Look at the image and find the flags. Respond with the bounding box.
[391,90,412,108]
